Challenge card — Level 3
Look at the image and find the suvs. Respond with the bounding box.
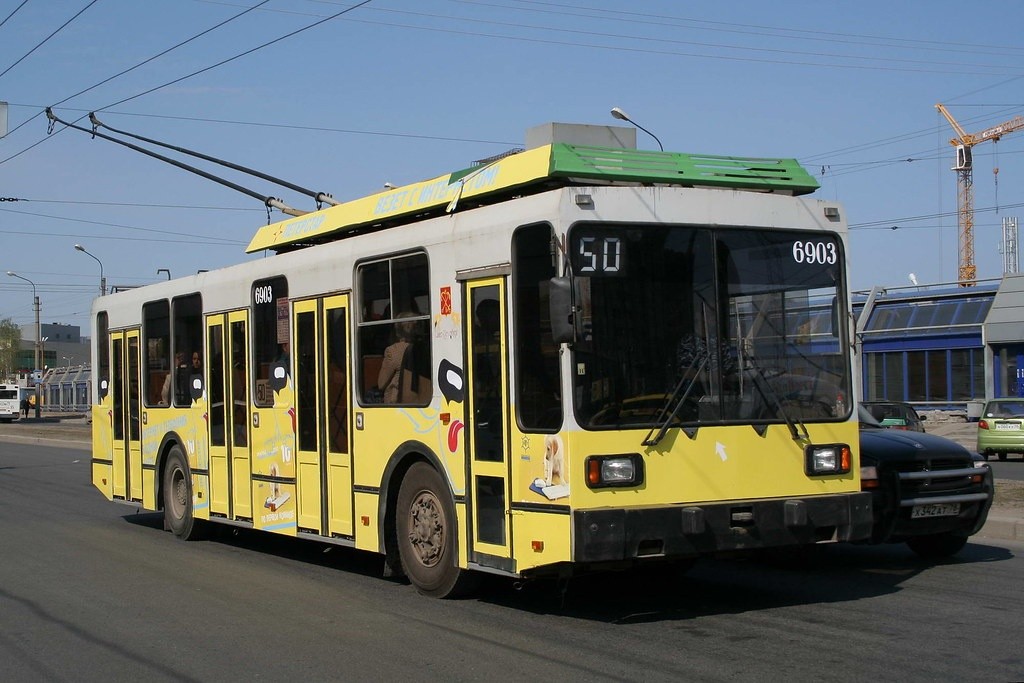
[859,400,927,432]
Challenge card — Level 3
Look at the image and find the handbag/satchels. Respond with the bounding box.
[364,389,384,403]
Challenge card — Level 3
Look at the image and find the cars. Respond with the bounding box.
[739,367,995,561]
[976,398,1024,461]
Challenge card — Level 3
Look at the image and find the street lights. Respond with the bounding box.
[610,108,664,152]
[41,336,48,379]
[6,271,40,420]
[62,356,74,366]
[74,244,105,297]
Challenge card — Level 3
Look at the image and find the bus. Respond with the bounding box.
[88,143,861,600]
[0,385,20,422]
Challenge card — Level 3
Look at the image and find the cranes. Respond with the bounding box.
[934,102,1024,287]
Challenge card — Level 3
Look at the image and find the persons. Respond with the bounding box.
[471,298,504,497]
[23,397,33,421]
[268,343,291,395]
[156,348,204,406]
[233,324,247,447]
[378,311,430,404]
